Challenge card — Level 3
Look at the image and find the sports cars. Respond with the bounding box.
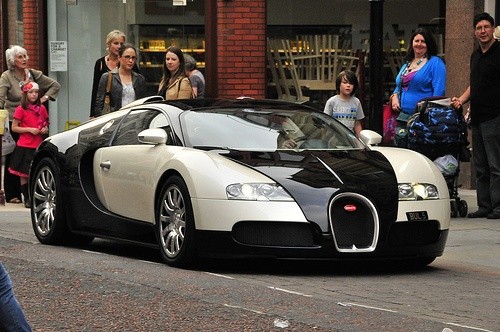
[27,92,454,274]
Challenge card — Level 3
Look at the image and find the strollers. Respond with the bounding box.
[394,96,471,217]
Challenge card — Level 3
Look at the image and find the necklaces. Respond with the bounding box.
[407,58,423,72]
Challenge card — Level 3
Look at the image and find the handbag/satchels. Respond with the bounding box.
[391,112,411,148]
[101,104,111,115]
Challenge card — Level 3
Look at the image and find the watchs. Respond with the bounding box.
[46,95,49,101]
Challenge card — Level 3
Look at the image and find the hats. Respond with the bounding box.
[19,79,39,92]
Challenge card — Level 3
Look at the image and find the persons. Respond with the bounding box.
[95,43,146,118]
[468,13,500,219]
[324,70,365,135]
[0,45,60,203]
[392,28,446,128]
[158,46,193,100]
[452,26,500,108]
[0,263,32,332]
[277,136,297,149]
[8,81,50,208]
[90,30,142,117]
[183,54,205,98]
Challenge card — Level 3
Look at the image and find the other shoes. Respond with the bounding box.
[9,197,22,205]
[468,211,500,219]
[24,201,30,208]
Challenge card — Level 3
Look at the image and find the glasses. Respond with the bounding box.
[123,55,137,62]
